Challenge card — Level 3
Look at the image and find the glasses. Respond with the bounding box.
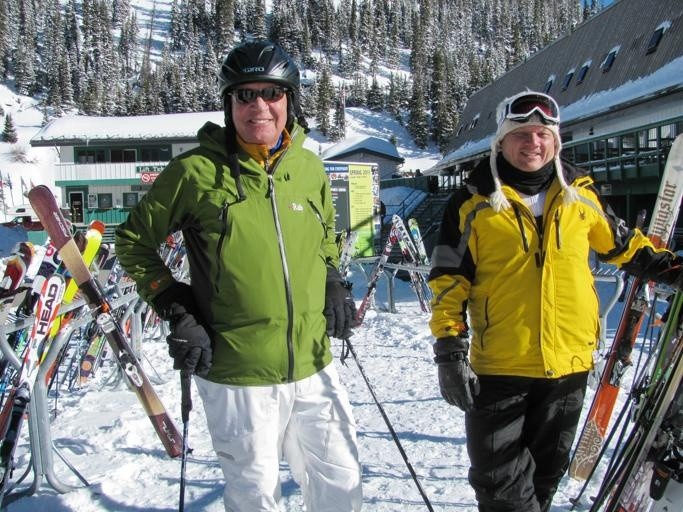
[506,93,560,124]
[230,84,291,103]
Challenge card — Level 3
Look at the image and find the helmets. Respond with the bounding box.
[219,41,300,97]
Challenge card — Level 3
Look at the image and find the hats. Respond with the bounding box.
[496,92,562,155]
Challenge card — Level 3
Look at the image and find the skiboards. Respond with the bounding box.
[568,133,682,511]
[392,214,435,313]
[340,226,398,326]
[0,229,102,505]
[26,184,191,458]
[79,229,190,380]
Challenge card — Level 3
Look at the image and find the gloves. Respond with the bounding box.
[154,283,215,378]
[325,266,356,338]
[434,336,481,414]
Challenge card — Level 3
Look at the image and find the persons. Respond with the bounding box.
[428,88,683,512]
[115,41,366,512]
